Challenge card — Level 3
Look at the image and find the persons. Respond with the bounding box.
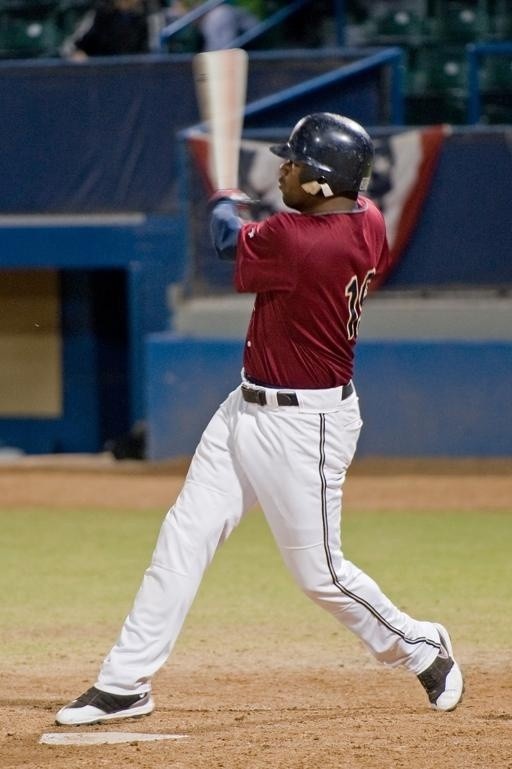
[56,1,148,65]
[55,111,466,724]
[162,0,261,54]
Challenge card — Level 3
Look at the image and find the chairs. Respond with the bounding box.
[0,0,512,120]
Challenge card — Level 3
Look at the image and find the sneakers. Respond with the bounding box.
[54,687,154,726]
[417,623,465,712]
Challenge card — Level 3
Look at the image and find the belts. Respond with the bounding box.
[241,383,353,406]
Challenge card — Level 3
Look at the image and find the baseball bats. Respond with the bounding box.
[193,49,248,192]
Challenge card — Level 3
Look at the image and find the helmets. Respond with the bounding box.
[269,112,375,193]
